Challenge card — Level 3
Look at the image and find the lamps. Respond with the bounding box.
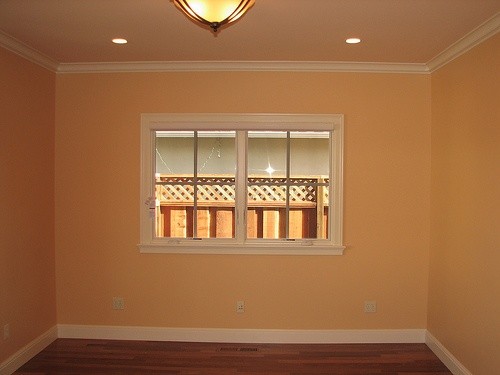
[170,0,256,32]
